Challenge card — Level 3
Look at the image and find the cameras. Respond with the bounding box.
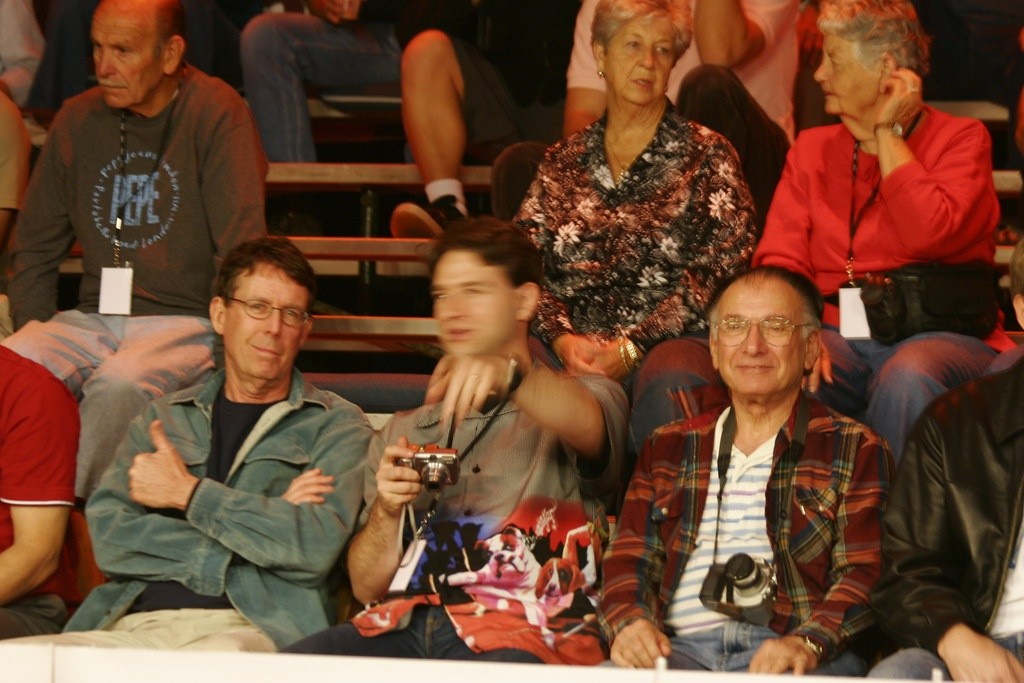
[699,554,778,626]
[397,444,459,492]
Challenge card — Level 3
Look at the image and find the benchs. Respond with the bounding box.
[57,116,1021,432]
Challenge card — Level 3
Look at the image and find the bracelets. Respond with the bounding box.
[616,332,641,377]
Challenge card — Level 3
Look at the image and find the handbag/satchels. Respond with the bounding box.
[860,260,1000,345]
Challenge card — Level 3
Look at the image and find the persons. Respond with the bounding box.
[491,1,807,255]
[596,265,897,675]
[748,0,1000,462]
[866,238,1024,683]
[524,0,757,383]
[1,0,636,673]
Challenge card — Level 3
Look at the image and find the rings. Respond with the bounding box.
[907,88,918,93]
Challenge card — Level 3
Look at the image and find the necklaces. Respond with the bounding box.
[605,139,628,184]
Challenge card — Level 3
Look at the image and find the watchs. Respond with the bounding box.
[802,636,823,661]
[874,121,904,137]
[501,354,531,401]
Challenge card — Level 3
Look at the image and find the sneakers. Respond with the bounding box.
[389,195,469,237]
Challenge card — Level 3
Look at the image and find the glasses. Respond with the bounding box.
[225,295,310,327]
[715,316,810,347]
